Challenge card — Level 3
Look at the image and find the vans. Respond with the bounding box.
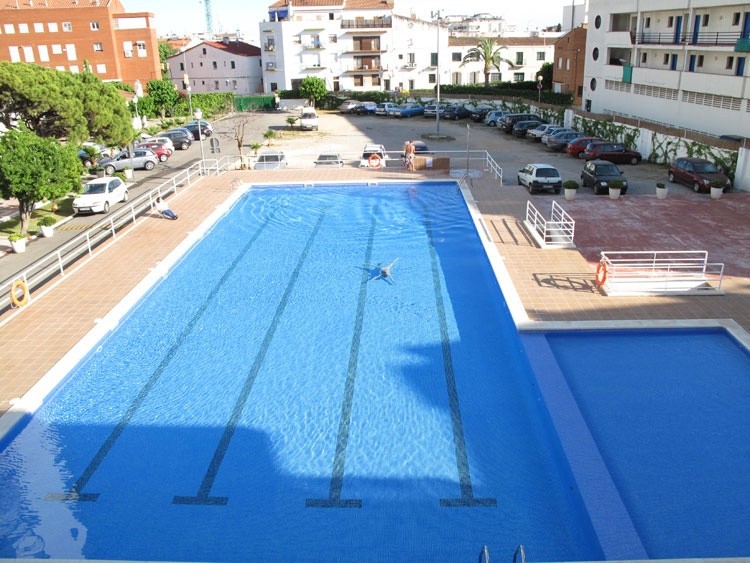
[298,107,320,131]
[500,113,551,134]
[403,141,435,165]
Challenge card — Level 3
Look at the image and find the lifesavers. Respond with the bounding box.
[595,261,608,286]
[369,154,381,167]
[10,280,27,305]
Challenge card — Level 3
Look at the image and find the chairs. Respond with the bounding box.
[153,196,177,220]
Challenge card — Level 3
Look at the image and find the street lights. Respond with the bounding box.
[537,76,543,104]
[187,85,193,118]
[194,107,207,174]
[132,95,139,118]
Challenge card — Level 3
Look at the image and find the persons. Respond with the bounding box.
[366,257,401,284]
[155,196,179,220]
[404,139,417,172]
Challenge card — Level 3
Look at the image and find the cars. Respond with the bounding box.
[313,152,345,168]
[335,99,513,127]
[513,119,610,160]
[517,163,563,195]
[99,148,159,174]
[253,152,287,170]
[73,176,130,215]
[667,157,732,194]
[56,120,215,162]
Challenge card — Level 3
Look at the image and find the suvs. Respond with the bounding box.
[583,142,643,166]
[580,160,629,194]
[358,144,386,167]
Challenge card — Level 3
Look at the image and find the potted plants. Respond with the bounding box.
[122,166,133,178]
[563,181,579,200]
[8,232,27,253]
[656,183,668,199]
[710,179,724,199]
[608,180,623,199]
[37,217,56,237]
